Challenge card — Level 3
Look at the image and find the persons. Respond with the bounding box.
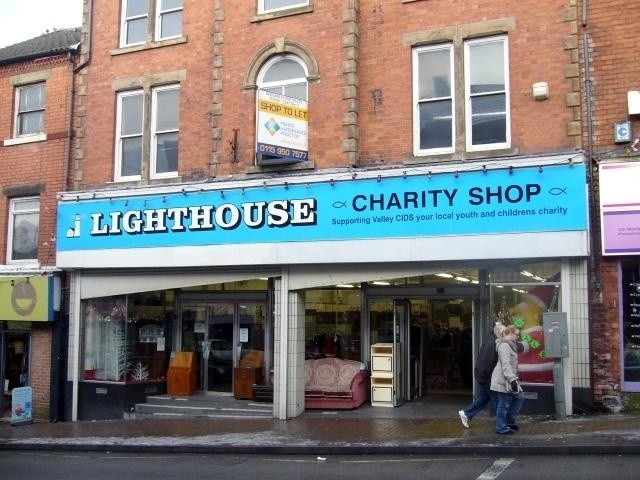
[458,322,530,427]
[489,325,525,435]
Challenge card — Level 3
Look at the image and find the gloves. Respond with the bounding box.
[511,381,518,391]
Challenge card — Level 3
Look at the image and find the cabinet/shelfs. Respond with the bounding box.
[370,342,400,407]
[253,382,274,402]
[167,351,196,396]
[234,356,263,399]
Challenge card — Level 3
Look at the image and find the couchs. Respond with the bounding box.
[305,356,370,411]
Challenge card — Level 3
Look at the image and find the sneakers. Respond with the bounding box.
[459,410,469,428]
